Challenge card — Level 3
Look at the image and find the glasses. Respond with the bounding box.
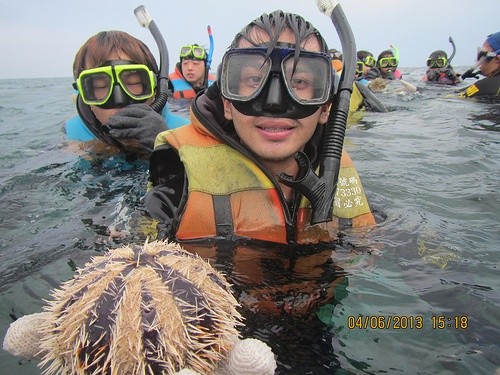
[220,45,332,120]
[75,61,160,110]
[378,58,398,69]
[363,56,376,67]
[356,61,365,74]
[180,46,207,62]
[329,52,342,60]
[476,50,494,62]
[426,57,447,67]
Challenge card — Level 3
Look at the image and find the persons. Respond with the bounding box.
[61,30,192,151]
[3,9,377,375]
[165,44,217,99]
[400,31,500,98]
[328,48,402,93]
[421,50,457,86]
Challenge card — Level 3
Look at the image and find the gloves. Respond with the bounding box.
[106,104,171,153]
[394,80,416,95]
[368,78,386,91]
